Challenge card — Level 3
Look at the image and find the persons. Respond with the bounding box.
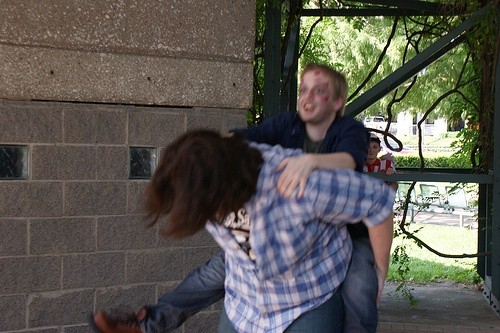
[140,126,396,333]
[363,131,399,191]
[89,60,381,333]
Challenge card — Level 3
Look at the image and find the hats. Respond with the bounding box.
[370,133,381,146]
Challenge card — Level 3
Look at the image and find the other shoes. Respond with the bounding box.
[89,306,147,333]
